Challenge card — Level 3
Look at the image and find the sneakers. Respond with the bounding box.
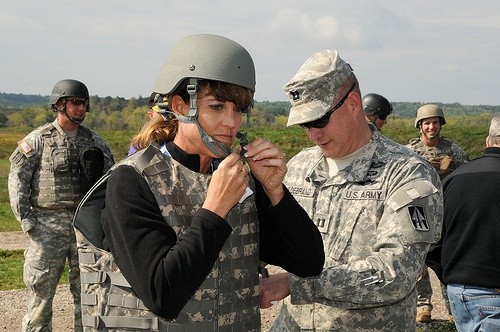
[415,305,432,323]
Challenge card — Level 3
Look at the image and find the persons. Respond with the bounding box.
[425,113,500,332]
[8,80,114,332]
[402,103,471,323]
[362,93,393,132]
[259,48,445,332]
[129,110,165,157]
[71,34,327,332]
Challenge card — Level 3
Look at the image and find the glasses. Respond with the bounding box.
[298,83,355,130]
[60,98,88,107]
[376,114,387,120]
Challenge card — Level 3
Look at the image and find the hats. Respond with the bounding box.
[284,48,355,127]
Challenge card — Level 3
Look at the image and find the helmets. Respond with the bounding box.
[362,92,392,118]
[153,34,256,96]
[50,80,90,113]
[415,104,446,128]
